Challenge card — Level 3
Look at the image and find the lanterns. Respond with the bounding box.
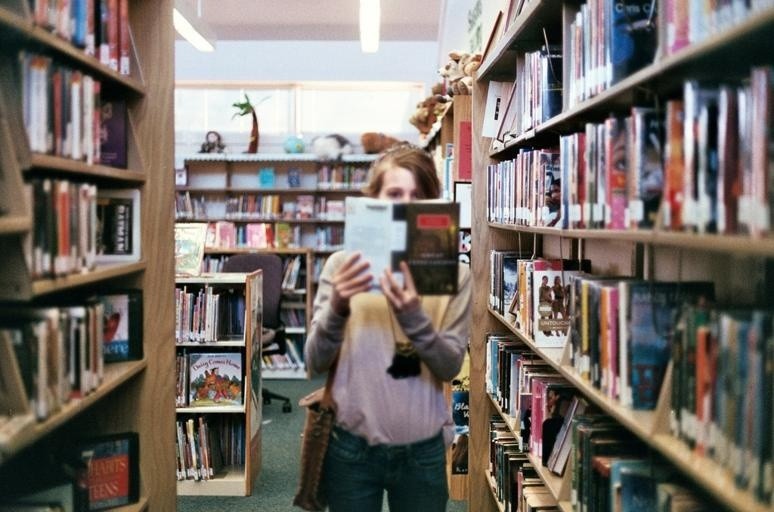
[309,132,351,163]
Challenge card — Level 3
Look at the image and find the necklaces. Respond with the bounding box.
[302,145,478,512]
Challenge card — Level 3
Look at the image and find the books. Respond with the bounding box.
[173,190,310,250]
[174,222,245,482]
[338,188,461,301]
[430,0,772,512]
[0,0,142,511]
[261,337,303,371]
[278,253,305,328]
[309,161,376,279]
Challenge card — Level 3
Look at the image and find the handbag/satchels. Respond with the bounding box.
[290,385,331,512]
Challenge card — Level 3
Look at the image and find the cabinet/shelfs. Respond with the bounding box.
[0,0,774,512]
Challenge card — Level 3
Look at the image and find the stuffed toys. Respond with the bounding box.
[409,52,480,137]
[356,130,410,155]
